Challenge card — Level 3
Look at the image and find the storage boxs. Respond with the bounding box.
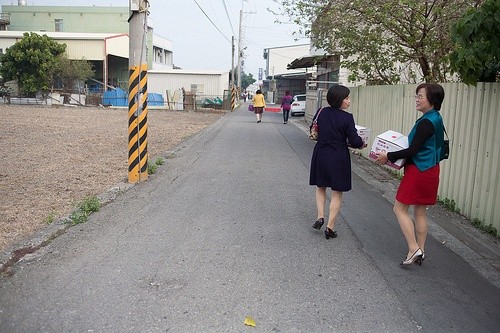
[349,124,371,148]
[368,130,408,171]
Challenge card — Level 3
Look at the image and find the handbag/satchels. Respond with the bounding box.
[442,125,449,159]
[248,102,254,111]
[309,107,324,140]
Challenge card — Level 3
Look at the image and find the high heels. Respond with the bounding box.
[400,248,425,267]
[312,218,324,230]
[325,228,337,239]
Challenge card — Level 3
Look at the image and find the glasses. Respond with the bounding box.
[415,95,427,99]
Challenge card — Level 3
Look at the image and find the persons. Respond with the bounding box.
[309,85,365,239]
[377,83,444,265]
[243,90,266,124]
[281,90,293,124]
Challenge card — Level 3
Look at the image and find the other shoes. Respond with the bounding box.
[283,121,287,124]
[257,120,261,123]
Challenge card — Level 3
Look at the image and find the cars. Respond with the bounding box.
[290,94,306,117]
